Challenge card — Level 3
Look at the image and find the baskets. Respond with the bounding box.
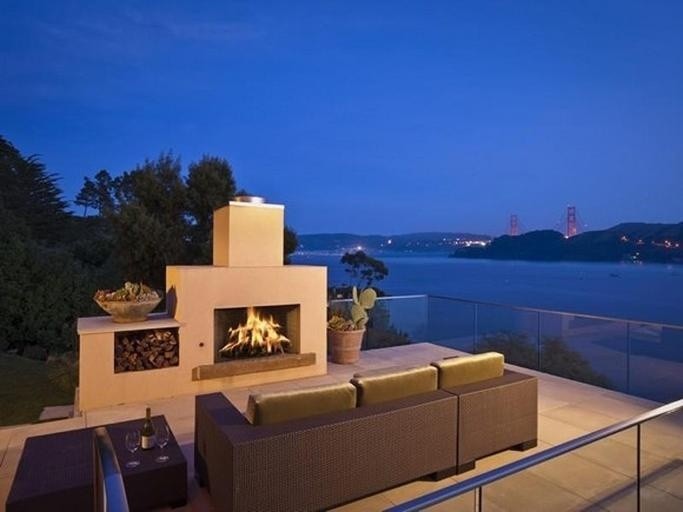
[93,292,164,323]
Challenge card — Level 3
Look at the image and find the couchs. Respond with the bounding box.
[192,349,539,512]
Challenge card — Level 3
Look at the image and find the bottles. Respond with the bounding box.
[141,407,155,450]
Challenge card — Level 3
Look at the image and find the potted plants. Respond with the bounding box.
[327,284,377,367]
[92,280,164,325]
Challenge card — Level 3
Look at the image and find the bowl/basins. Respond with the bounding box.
[94,298,163,322]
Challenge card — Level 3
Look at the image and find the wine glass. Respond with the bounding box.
[125,431,141,466]
[155,425,170,464]
